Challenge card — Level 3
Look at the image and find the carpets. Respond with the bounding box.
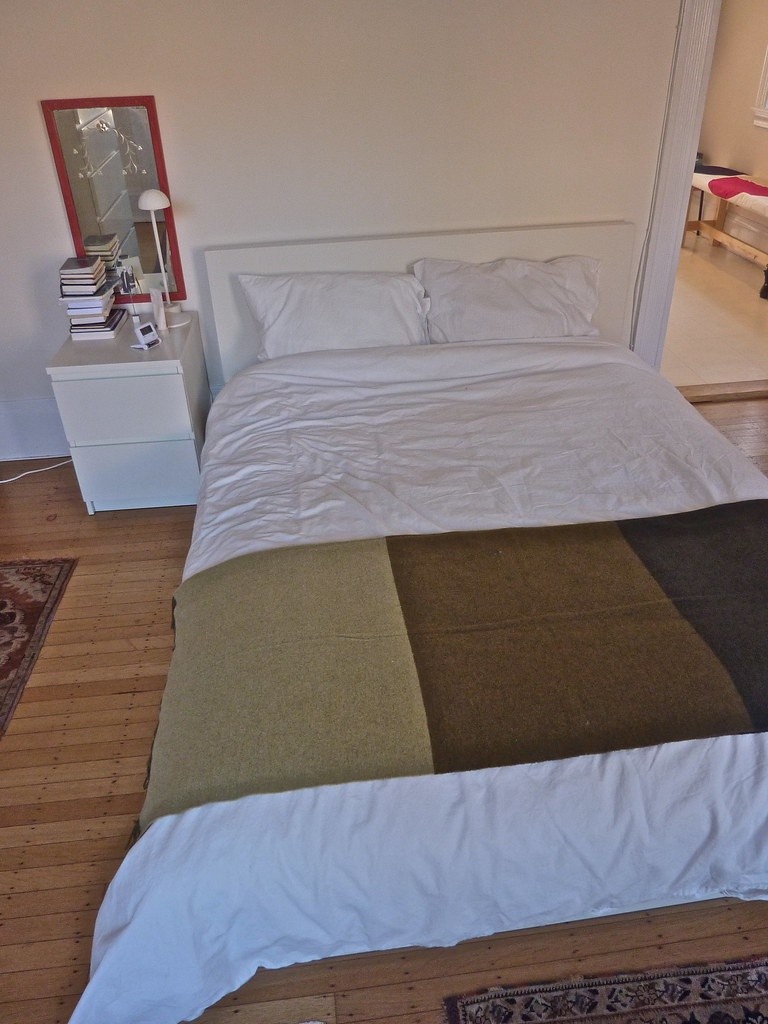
[443,953,768,1024]
[0,558,80,742]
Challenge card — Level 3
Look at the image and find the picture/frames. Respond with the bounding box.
[40,95,187,304]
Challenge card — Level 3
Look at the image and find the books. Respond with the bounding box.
[83,233,126,277]
[59,255,128,340]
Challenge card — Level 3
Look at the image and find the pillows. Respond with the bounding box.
[237,271,431,362]
[411,255,600,345]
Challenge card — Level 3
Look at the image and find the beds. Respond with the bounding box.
[68,220,768,1024]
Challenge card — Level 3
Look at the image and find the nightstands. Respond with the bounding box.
[46,311,212,515]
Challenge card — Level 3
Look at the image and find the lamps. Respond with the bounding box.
[138,189,182,313]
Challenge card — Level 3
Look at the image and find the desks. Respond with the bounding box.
[681,166,768,268]
